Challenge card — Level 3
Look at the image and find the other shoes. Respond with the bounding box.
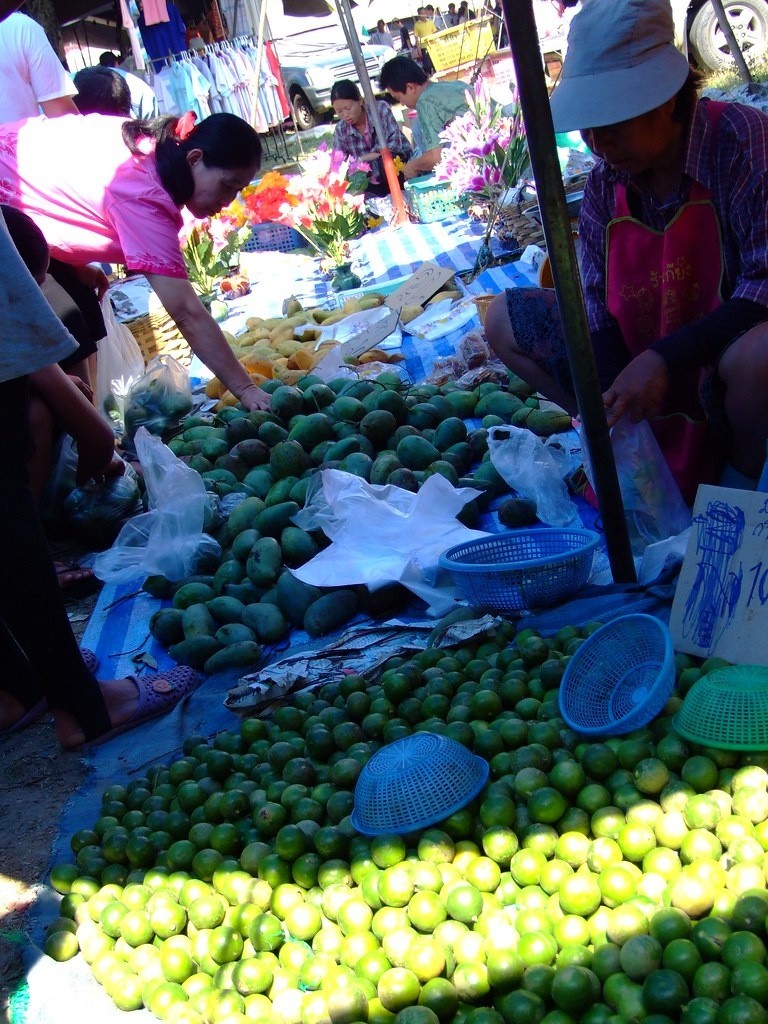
[57,556,103,602]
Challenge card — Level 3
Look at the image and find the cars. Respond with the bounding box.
[263,16,401,131]
[668,0,768,82]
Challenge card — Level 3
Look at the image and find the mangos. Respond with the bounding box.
[200,290,463,414]
[52,376,572,672]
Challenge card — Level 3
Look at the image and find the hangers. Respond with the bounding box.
[164,34,249,67]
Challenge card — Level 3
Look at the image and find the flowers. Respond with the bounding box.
[176,142,382,293]
[431,72,529,217]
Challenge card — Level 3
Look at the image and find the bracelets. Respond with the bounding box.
[358,157,363,163]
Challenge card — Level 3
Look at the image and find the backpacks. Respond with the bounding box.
[74,69,123,110]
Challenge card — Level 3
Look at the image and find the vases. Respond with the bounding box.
[331,262,361,291]
[221,267,250,300]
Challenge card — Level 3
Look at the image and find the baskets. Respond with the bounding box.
[559,612,677,736]
[242,223,302,252]
[349,732,491,835]
[671,664,768,752]
[438,527,602,611]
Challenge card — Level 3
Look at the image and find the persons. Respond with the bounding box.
[485,0,767,508]
[330,55,474,198]
[368,0,508,74]
[0,0,272,752]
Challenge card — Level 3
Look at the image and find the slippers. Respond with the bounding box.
[78,665,198,752]
[3,647,100,737]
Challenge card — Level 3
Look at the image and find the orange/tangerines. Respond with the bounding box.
[41,621,768,1024]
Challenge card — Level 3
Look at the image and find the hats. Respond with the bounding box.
[547,0,692,133]
[100,52,125,67]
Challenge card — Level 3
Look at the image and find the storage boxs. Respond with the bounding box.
[405,174,466,224]
[243,216,307,255]
[419,15,493,71]
